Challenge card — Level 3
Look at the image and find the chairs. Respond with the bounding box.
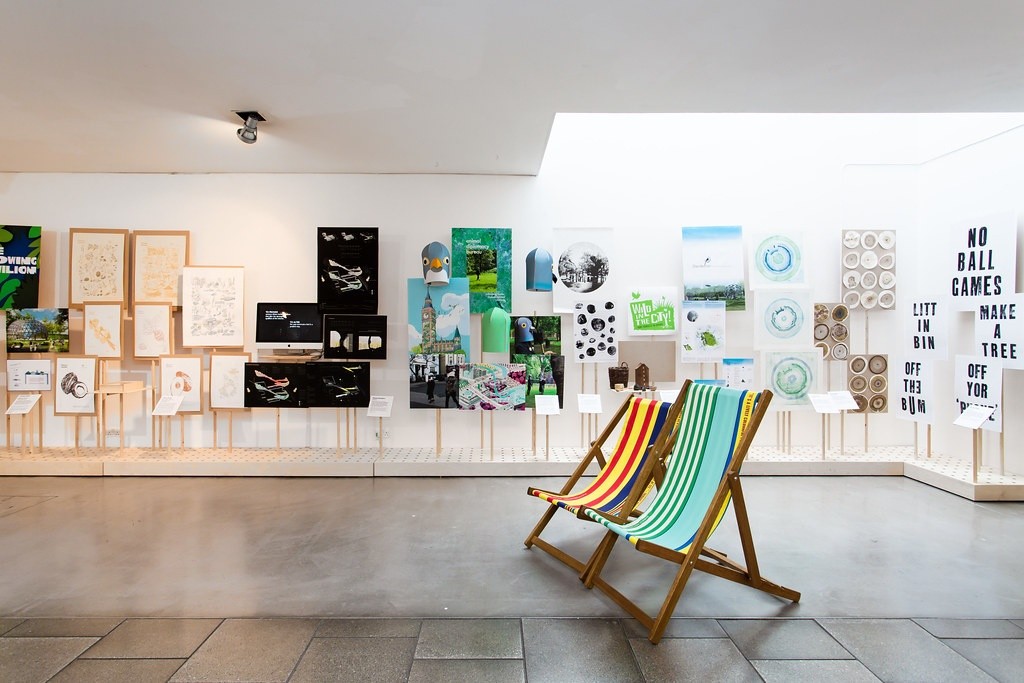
[524,392,728,587]
[583,379,802,646]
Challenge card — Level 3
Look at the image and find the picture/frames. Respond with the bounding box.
[4,227,252,416]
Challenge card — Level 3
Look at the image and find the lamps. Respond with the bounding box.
[231,108,272,144]
